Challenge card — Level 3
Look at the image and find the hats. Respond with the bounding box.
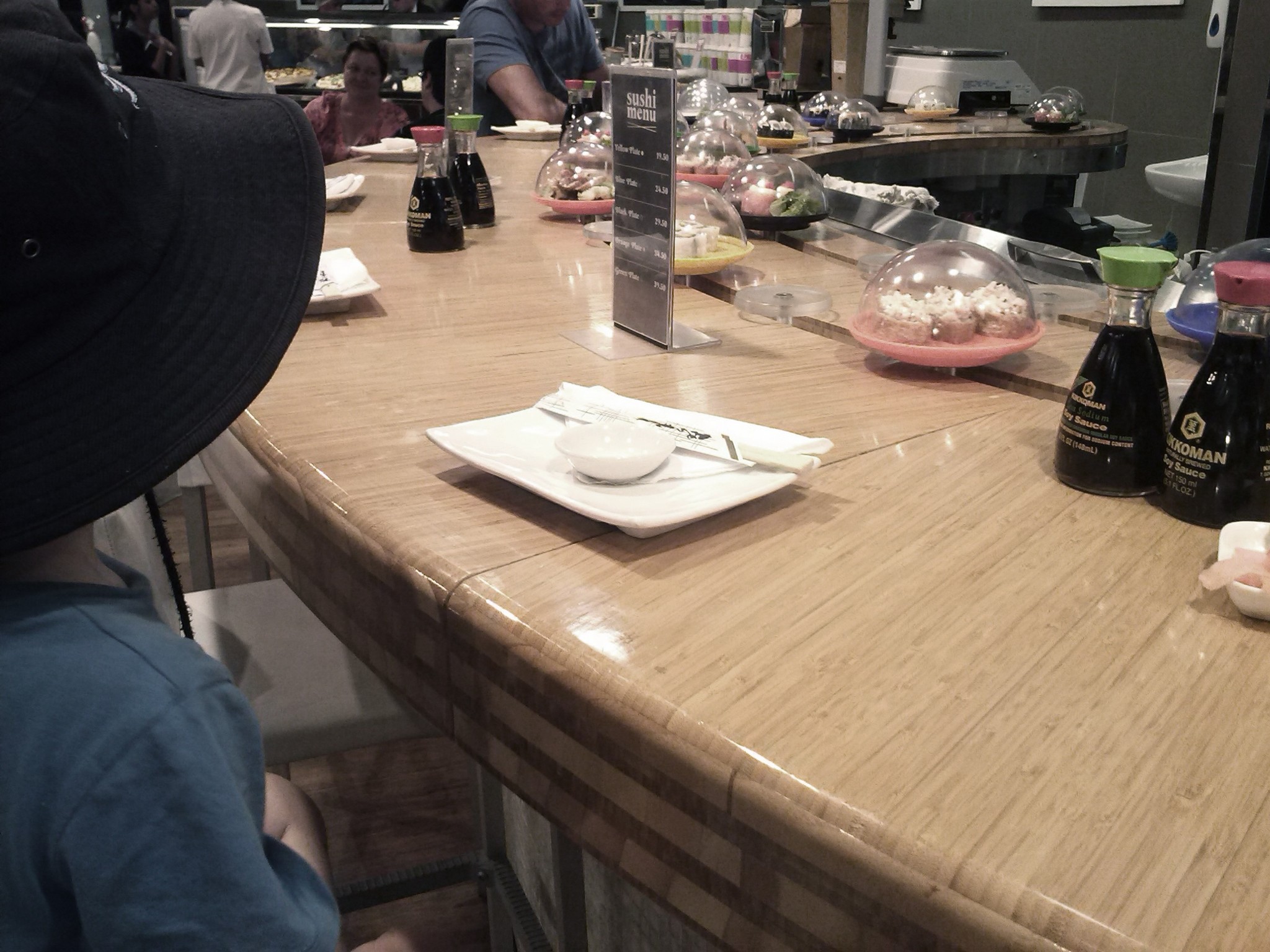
[0,0,327,556]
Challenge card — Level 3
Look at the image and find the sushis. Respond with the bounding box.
[550,90,1079,344]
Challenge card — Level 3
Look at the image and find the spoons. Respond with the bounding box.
[1198,546,1270,590]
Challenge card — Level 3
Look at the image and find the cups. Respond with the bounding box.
[640,7,753,87]
[601,47,625,65]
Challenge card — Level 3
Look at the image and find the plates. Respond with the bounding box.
[350,144,425,162]
[299,247,381,315]
[324,174,366,212]
[1163,301,1219,351]
[489,125,564,140]
[515,120,549,129]
[381,137,416,150]
[1022,113,1081,134]
[530,64,964,293]
[428,391,818,538]
[850,316,1047,371]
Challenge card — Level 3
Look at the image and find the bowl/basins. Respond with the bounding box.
[554,419,676,482]
[1218,521,1270,620]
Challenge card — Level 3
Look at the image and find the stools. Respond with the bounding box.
[180,576,494,952]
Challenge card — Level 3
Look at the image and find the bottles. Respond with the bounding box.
[1158,263,1270,532]
[762,72,784,107]
[407,125,467,254]
[1052,241,1177,497]
[445,113,497,229]
[781,73,802,116]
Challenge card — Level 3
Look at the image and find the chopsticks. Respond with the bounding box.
[535,392,814,472]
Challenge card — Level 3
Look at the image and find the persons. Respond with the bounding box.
[453,0,614,135]
[0,0,457,952]
